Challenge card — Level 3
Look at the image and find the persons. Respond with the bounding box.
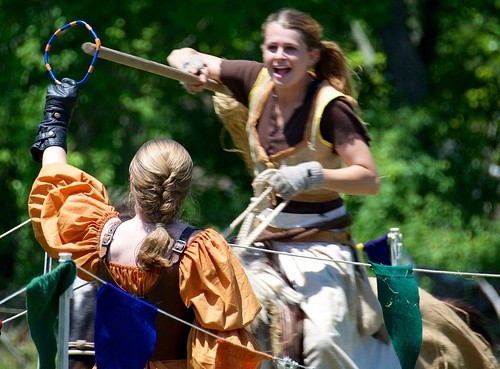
[166,8,380,369]
[28,78,263,369]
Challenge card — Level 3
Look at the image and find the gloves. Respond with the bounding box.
[31,78,78,163]
